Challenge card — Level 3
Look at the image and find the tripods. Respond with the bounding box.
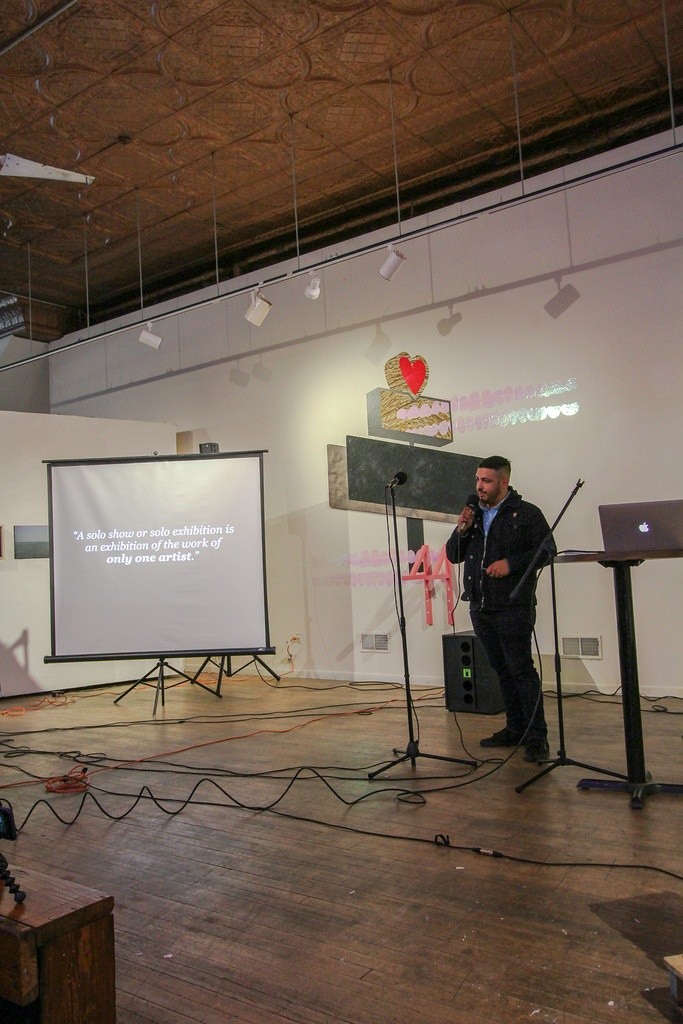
[509,478,628,793]
[368,487,478,781]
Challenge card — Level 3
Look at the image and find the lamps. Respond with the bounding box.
[380,245,406,280]
[244,290,272,326]
[139,325,162,350]
[306,279,320,299]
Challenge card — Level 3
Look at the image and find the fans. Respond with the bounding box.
[0,154,95,185]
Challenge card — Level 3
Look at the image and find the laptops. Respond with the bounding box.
[598,499,683,553]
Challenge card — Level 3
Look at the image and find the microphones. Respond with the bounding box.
[460,494,480,531]
[385,472,407,489]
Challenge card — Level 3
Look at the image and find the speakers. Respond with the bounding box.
[442,629,505,715]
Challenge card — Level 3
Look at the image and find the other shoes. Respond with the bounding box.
[524,736,550,762]
[480,727,526,747]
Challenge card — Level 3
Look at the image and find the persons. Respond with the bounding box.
[446,456,557,763]
